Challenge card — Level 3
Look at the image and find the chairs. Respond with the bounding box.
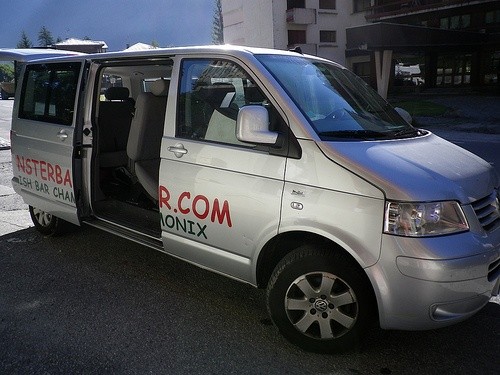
[97,79,170,209]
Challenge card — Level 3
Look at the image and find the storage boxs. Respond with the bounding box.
[203,107,258,148]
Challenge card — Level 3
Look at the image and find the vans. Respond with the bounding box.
[0,45,500,355]
